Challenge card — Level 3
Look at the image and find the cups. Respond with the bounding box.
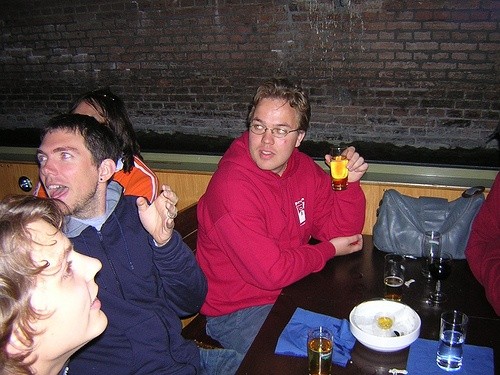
[330,145,349,191]
[436,311,469,371]
[307,327,334,375]
[383,253,405,287]
[421,231,442,278]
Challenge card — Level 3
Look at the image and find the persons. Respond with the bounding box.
[1,193,108,375]
[463,126,500,320]
[35,113,244,375]
[196,79,369,357]
[33,85,160,205]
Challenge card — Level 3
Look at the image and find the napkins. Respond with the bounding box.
[270,305,358,366]
[405,338,494,375]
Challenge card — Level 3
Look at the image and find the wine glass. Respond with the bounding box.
[426,250,453,303]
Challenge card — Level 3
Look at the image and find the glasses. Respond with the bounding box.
[91,89,114,101]
[249,124,300,137]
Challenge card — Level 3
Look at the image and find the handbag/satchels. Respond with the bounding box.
[373,187,485,260]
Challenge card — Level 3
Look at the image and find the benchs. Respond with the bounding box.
[173,201,223,348]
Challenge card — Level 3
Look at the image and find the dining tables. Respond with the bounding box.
[234,235,499,375]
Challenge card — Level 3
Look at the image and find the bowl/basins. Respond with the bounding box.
[349,299,421,353]
